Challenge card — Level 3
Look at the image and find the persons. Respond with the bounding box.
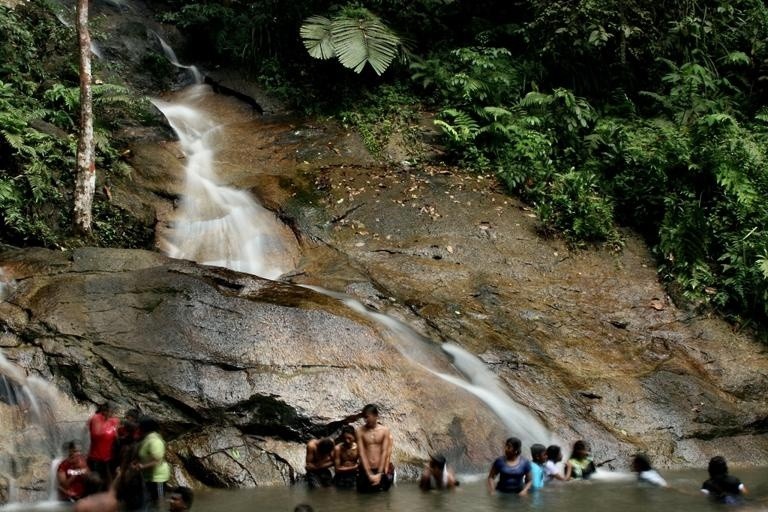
[418,453,456,496]
[305,404,395,497]
[627,453,672,493]
[670,455,751,507]
[525,443,547,493]
[542,444,573,487]
[486,437,532,506]
[564,440,595,481]
[56,400,194,512]
